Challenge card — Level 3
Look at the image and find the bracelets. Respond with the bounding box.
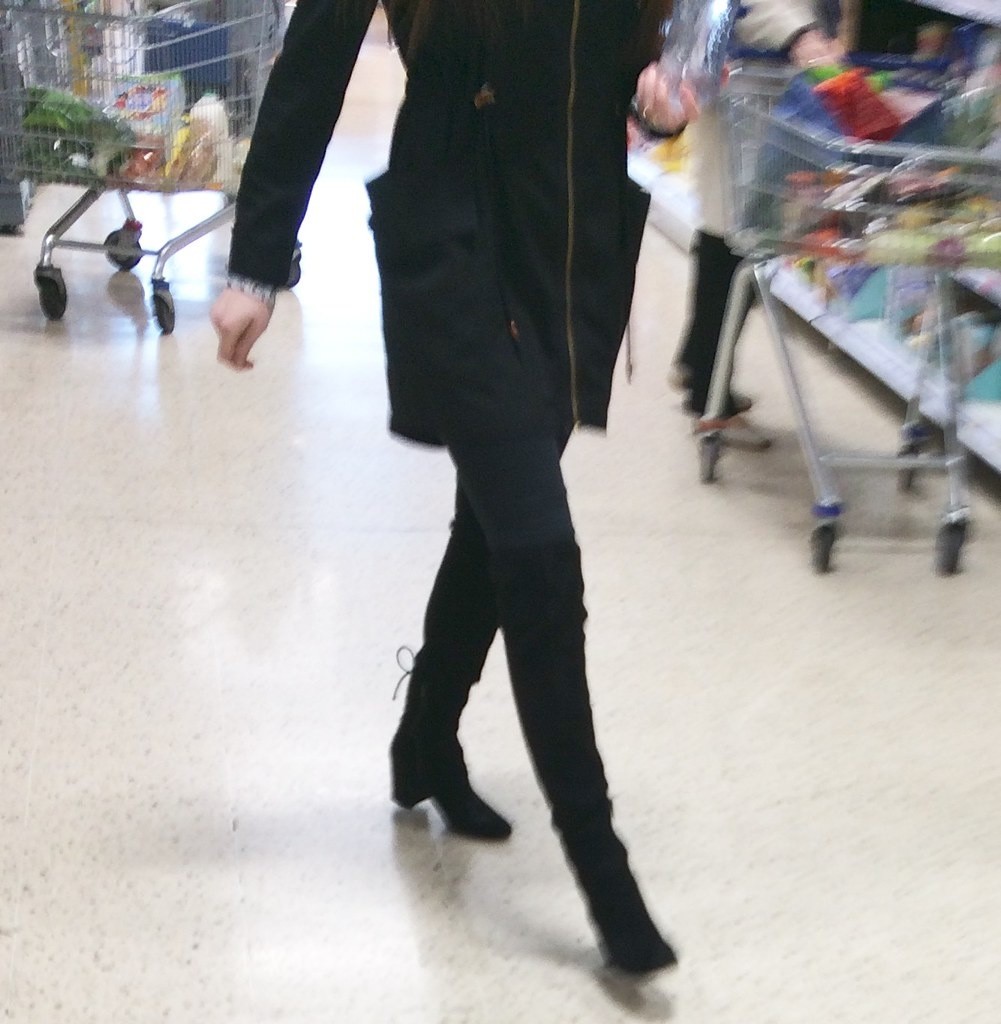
[227,272,275,305]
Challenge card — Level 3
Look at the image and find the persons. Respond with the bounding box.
[210,0,691,977]
[669,0,839,449]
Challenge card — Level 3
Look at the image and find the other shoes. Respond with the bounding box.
[676,374,754,414]
[691,410,770,452]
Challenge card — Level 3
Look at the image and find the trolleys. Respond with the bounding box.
[1,1,304,333]
[691,44,1000,576]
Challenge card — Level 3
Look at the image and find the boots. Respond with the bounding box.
[504,584,678,976]
[392,520,514,841]
